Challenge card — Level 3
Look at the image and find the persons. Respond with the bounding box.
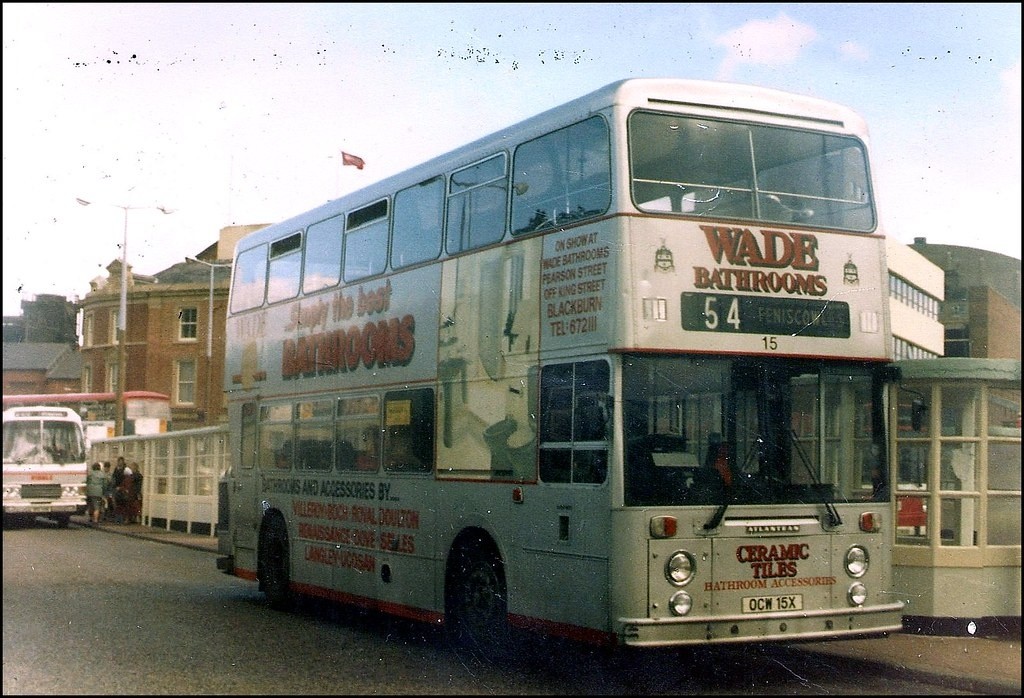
[85,457,143,526]
[355,427,383,472]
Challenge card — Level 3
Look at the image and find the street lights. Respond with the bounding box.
[75,196,175,439]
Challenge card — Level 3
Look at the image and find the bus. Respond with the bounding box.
[213,74,929,661]
[3,389,172,440]
[3,403,89,528]
